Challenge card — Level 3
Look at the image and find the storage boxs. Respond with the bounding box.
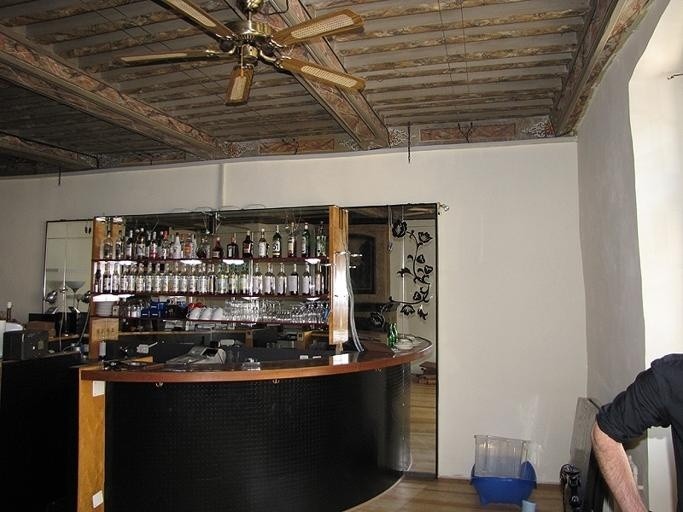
[473,435,528,479]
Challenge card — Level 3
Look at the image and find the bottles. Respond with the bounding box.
[130,305,142,318]
[386,322,398,347]
[92,222,327,297]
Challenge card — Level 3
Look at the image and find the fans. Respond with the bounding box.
[120,0,366,106]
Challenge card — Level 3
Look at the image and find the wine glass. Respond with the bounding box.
[290,301,329,325]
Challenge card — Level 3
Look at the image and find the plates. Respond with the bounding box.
[394,344,412,350]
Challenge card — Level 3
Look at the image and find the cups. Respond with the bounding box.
[188,300,281,322]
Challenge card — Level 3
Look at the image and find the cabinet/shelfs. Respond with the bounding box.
[91,206,349,346]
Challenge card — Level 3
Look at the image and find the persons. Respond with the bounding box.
[590,354,683,512]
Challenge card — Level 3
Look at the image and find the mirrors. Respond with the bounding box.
[43,219,93,314]
[343,203,438,481]
